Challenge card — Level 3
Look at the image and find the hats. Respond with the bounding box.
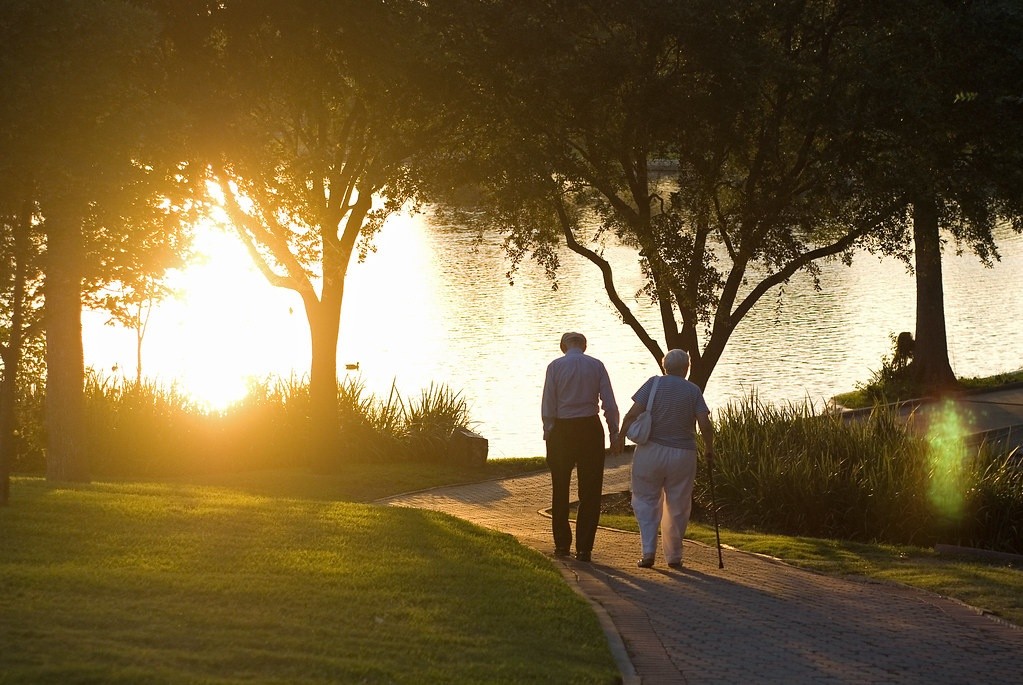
[561,332,587,354]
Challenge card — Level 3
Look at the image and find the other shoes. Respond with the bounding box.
[553,545,570,556]
[668,561,683,567]
[575,550,591,561]
[637,560,654,567]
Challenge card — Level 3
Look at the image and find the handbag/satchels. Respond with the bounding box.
[626,375,660,444]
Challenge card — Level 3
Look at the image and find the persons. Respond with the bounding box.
[619,349,713,567]
[542,332,619,562]
